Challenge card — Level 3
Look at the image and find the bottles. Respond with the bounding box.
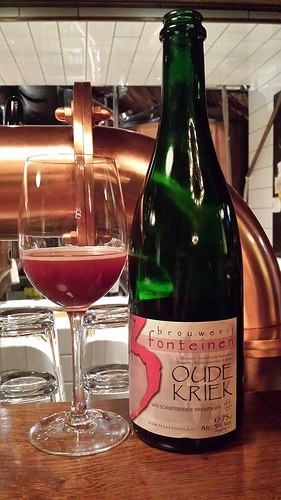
[127,8,247,455]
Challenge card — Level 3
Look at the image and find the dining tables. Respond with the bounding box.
[0,398,281,500]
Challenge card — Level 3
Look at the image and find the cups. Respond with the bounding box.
[80,306,130,397]
[1,307,58,405]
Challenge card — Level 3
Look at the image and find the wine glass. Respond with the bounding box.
[18,151,130,457]
